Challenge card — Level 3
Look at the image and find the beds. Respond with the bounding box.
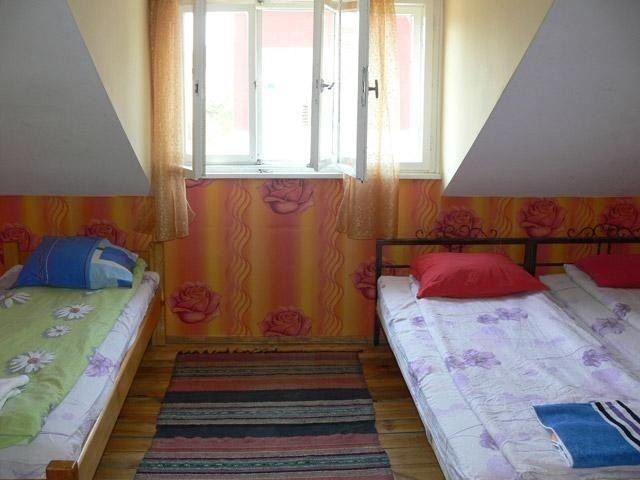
[1,242,166,480]
[375,237,640,477]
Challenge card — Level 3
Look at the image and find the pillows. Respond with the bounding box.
[9,236,139,289]
[410,252,553,301]
[575,252,638,289]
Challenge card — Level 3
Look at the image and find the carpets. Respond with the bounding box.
[133,346,395,480]
